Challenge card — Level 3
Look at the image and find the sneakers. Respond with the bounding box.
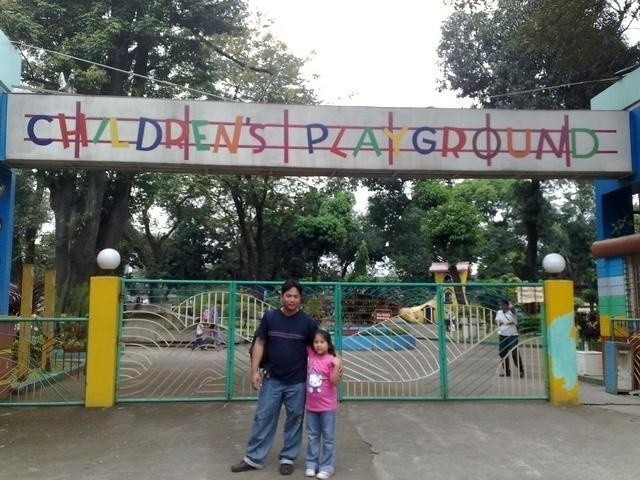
[499,370,524,378]
[305,468,316,477]
[280,464,294,475]
[231,460,257,473]
[315,470,333,479]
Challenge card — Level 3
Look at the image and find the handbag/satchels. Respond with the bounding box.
[248,309,275,367]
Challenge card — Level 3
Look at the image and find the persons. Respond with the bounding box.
[494,299,525,378]
[190,318,208,351]
[232,279,343,476]
[208,300,222,346]
[305,330,341,480]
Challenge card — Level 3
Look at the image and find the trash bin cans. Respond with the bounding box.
[603,341,634,395]
